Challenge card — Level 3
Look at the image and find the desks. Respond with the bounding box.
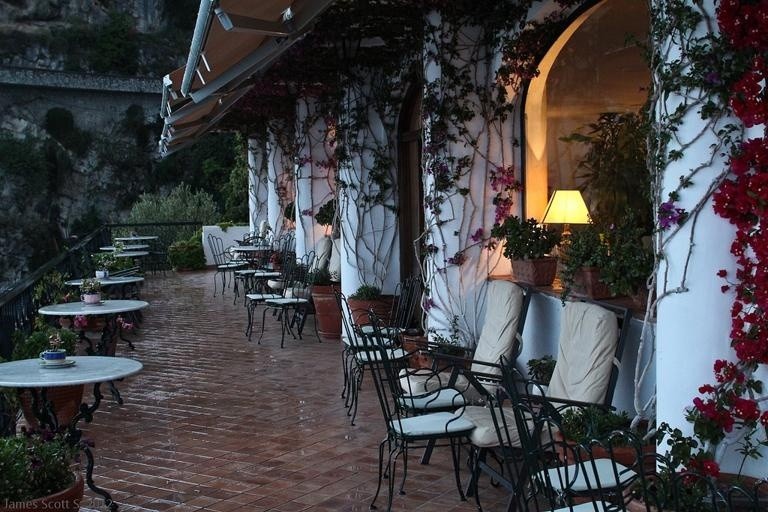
[0,235,159,511]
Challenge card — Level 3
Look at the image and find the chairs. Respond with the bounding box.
[207,232,768,512]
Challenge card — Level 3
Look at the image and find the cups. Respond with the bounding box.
[96,270,109,279]
[39,349,67,365]
[79,291,101,304]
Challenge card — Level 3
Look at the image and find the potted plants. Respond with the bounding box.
[489,216,656,310]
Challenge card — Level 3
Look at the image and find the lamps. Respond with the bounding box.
[540,188,595,264]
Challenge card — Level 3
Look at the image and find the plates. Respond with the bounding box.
[36,359,76,369]
[80,301,104,307]
